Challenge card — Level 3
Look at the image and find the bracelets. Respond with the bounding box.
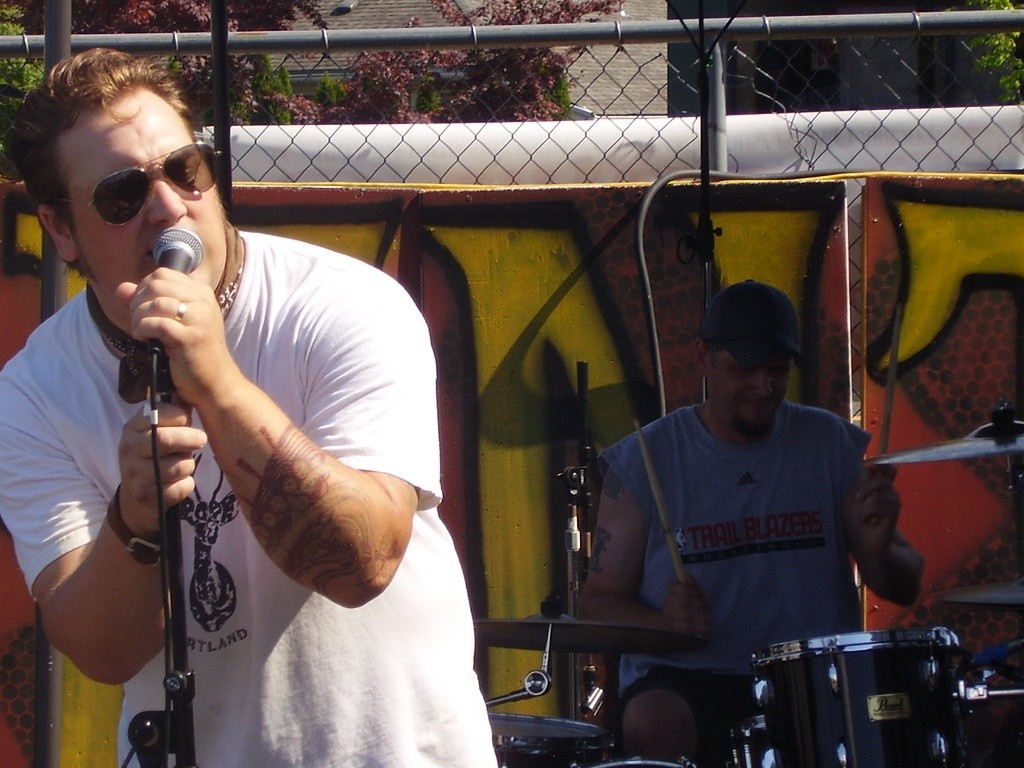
[107,481,162,567]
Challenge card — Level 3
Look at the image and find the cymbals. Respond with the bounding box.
[472,593,713,659]
[861,394,1023,468]
[932,574,1024,607]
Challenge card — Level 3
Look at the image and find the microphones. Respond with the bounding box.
[146,227,205,366]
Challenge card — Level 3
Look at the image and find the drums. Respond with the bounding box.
[486,711,615,768]
[565,755,697,768]
[748,623,972,768]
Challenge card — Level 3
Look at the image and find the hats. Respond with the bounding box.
[704,278,806,368]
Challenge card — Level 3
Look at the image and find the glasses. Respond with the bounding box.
[51,141,222,227]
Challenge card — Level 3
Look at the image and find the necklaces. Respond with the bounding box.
[85,221,243,356]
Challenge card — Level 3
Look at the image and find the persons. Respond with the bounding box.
[578,279,924,763]
[0,45,501,768]
